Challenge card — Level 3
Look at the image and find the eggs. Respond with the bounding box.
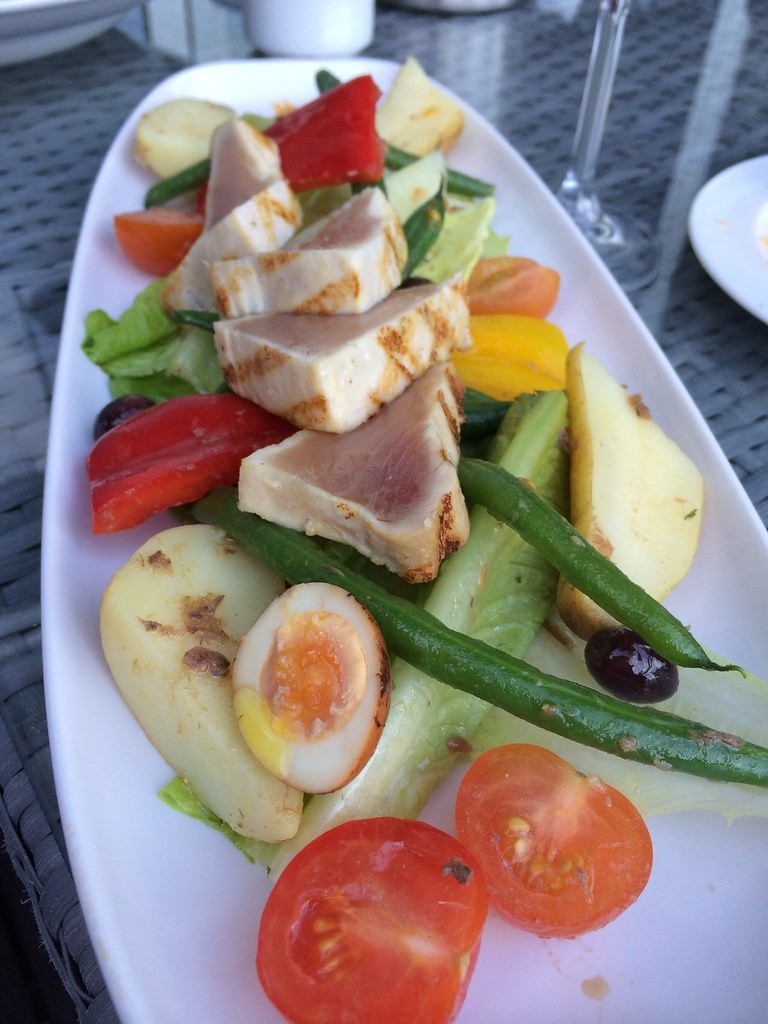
[231,581,390,794]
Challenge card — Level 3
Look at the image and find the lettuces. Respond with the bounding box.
[156,391,573,887]
[462,599,767,820]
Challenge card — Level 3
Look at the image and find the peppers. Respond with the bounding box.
[86,74,569,534]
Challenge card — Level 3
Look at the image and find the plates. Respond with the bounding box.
[687,151,766,331]
[37,56,766,1023]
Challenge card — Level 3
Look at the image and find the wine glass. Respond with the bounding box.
[548,0,663,297]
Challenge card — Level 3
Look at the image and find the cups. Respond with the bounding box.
[244,0,374,58]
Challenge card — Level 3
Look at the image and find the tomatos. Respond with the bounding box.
[256,742,654,1023]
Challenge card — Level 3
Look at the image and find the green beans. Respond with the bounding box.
[190,483,768,789]
[458,455,755,681]
